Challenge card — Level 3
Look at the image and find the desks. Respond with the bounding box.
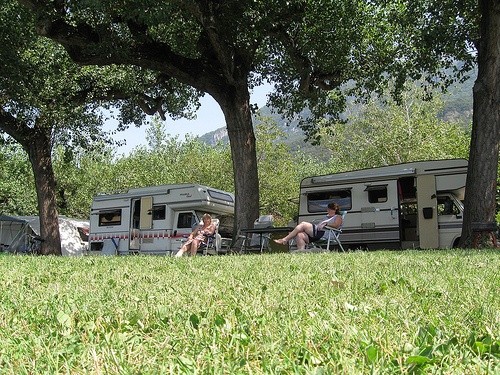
[240,227,298,253]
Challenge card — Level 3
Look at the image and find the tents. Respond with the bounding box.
[0,213,90,256]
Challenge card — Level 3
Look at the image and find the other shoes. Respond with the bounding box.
[273,239,287,246]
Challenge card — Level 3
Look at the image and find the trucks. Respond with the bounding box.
[83,182,234,254]
[288,157,469,251]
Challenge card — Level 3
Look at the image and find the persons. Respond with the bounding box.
[273,203,342,250]
[175,213,216,256]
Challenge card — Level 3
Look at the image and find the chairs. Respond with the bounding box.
[312,210,347,253]
[239,215,273,254]
[176,218,222,255]
